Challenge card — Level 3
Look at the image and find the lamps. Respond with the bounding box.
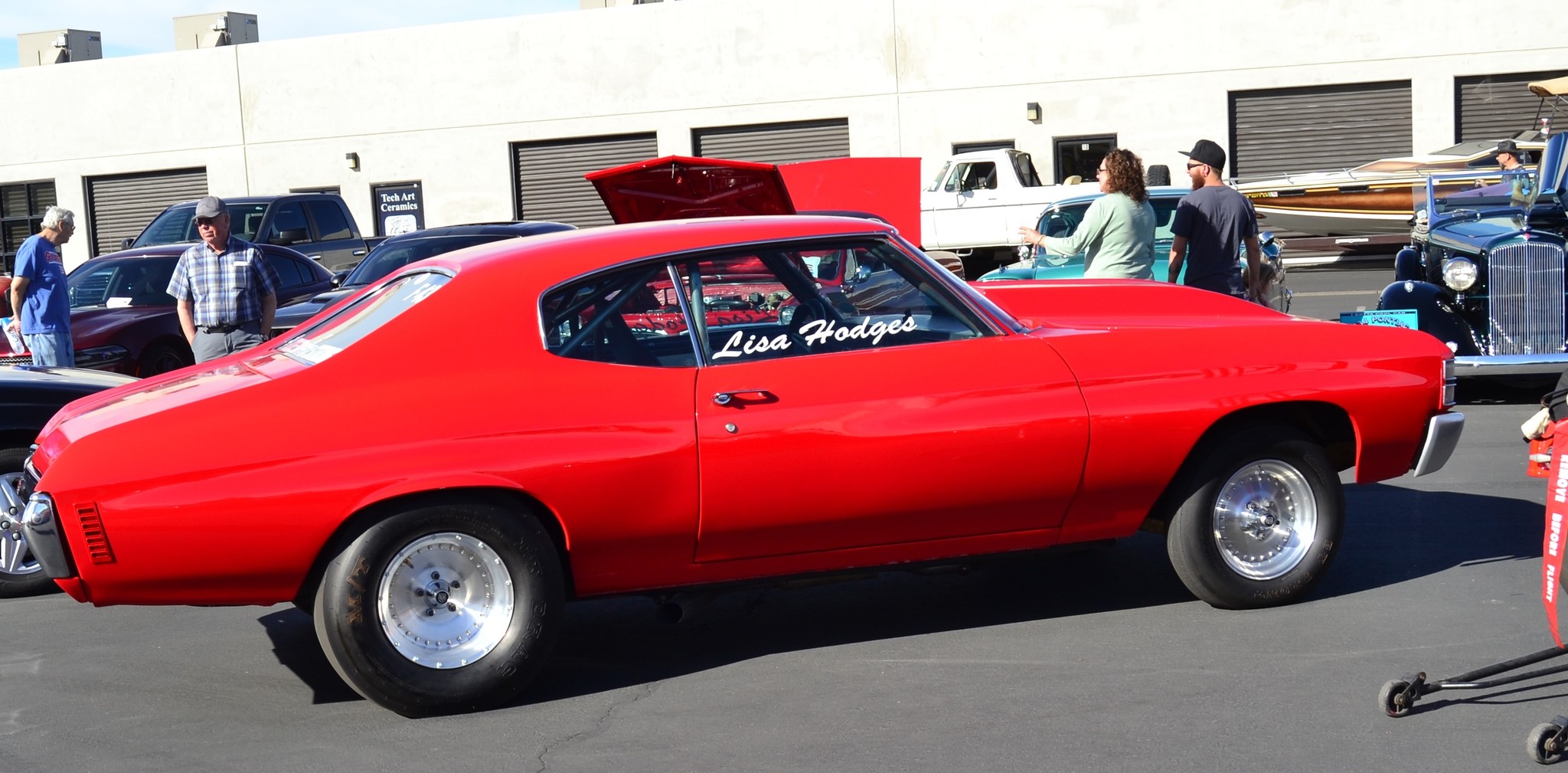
[1027,102,1038,120]
[346,153,358,169]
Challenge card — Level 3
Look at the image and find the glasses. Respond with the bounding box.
[1187,162,1204,171]
[195,215,223,226]
[1096,168,1107,174]
[63,221,76,231]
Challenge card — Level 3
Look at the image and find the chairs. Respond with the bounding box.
[248,213,293,232]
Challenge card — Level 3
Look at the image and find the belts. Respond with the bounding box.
[207,323,242,334]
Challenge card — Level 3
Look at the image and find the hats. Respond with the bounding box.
[1178,140,1225,171]
[191,196,228,220]
[1492,140,1523,152]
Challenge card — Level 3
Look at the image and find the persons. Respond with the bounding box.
[7,206,76,367]
[1018,149,1156,281]
[1168,140,1260,301]
[1475,140,1530,187]
[1242,263,1277,310]
[165,196,277,364]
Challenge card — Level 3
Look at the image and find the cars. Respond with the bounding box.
[1375,129,1568,396]
[0,360,158,597]
[264,220,575,339]
[0,241,346,378]
[974,185,1295,318]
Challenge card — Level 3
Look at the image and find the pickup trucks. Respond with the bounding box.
[123,191,410,283]
[919,147,1102,257]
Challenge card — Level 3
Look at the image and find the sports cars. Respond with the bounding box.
[17,154,1469,722]
